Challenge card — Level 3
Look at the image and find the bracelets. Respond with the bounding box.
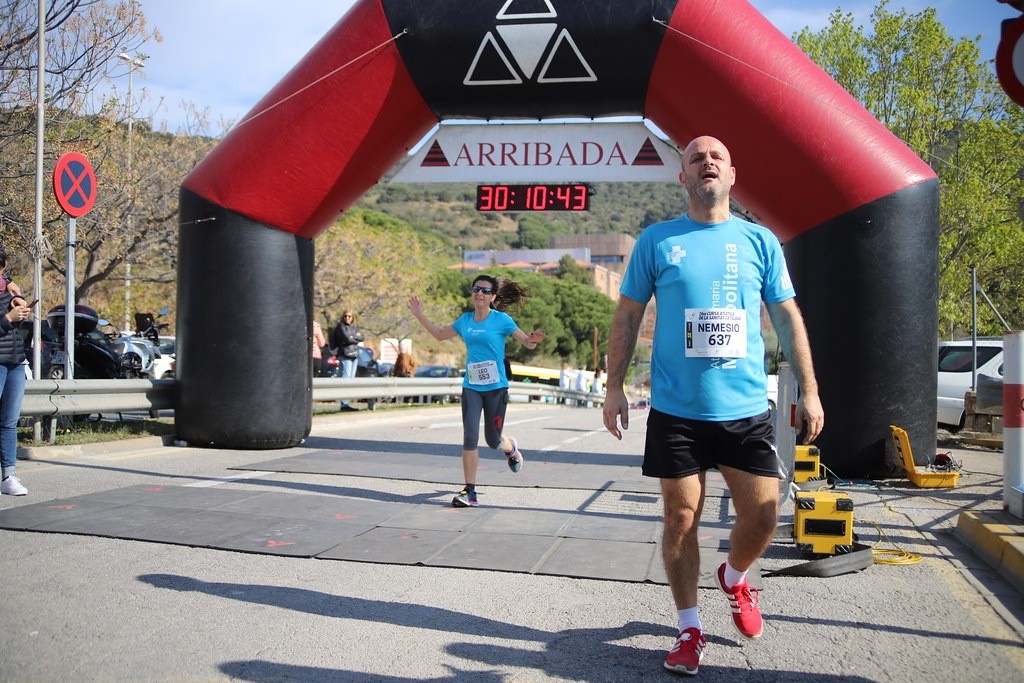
[531,342,537,344]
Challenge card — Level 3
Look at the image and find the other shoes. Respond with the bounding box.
[1,476,27,496]
[341,404,359,413]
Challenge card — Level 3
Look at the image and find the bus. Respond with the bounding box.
[510,364,627,408]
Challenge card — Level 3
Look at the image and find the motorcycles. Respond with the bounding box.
[48,303,169,379]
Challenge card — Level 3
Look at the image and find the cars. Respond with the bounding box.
[630,400,650,409]
[414,366,467,403]
[118,331,179,379]
[316,346,396,377]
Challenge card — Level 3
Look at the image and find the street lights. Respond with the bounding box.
[116,50,146,331]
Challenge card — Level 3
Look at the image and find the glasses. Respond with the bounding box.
[344,315,351,318]
[472,286,493,295]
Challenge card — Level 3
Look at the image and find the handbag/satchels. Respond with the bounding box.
[327,322,347,351]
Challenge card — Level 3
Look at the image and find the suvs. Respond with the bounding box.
[12,319,57,377]
[935,336,1005,433]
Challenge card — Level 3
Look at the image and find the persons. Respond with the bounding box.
[393,345,417,403]
[336,310,365,411]
[406,274,548,507]
[560,363,602,406]
[0,245,30,495]
[603,136,824,674]
[313,313,325,377]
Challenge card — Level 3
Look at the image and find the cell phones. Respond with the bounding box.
[26,299,39,308]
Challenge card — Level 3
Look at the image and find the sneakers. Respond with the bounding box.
[452,483,478,507]
[663,627,706,675]
[713,562,764,641]
[507,436,523,473]
